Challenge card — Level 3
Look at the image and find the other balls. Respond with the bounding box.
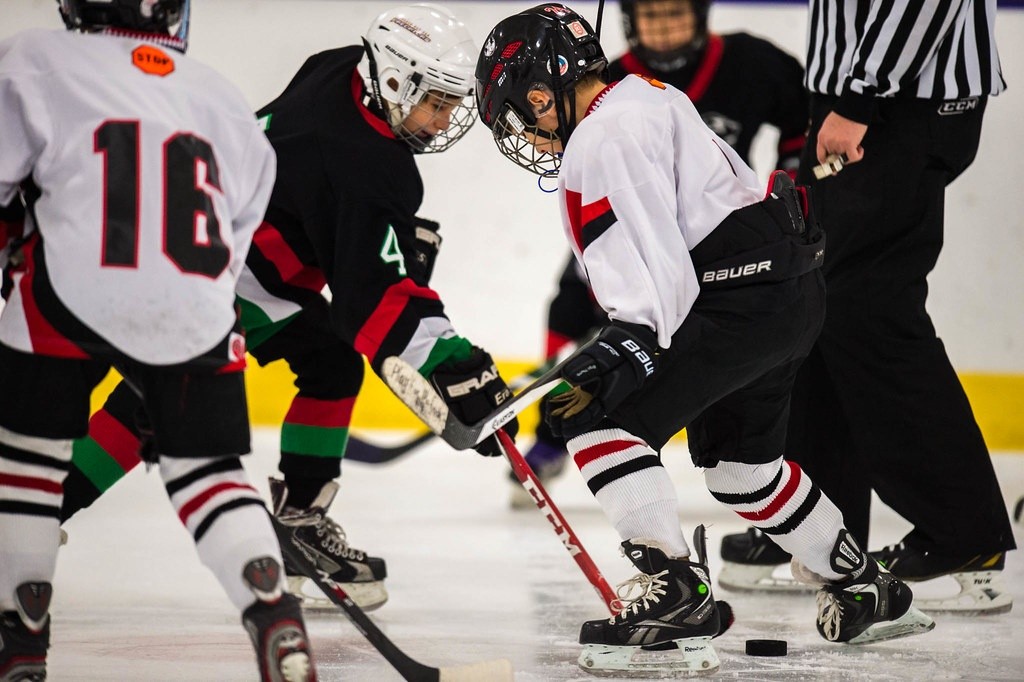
[743,637,788,658]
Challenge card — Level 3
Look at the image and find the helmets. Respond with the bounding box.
[475,3,609,179]
[356,4,481,154]
[619,0,709,85]
[54,0,191,52]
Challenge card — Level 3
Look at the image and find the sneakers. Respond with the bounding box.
[816,527,937,645]
[720,525,824,598]
[510,442,569,510]
[267,476,389,614]
[578,525,722,678]
[241,555,319,682]
[0,581,53,682]
[869,525,1013,618]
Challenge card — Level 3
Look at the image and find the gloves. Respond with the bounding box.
[539,325,666,440]
[422,345,519,458]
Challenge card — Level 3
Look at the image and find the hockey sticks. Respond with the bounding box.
[382,332,736,652]
[265,505,442,682]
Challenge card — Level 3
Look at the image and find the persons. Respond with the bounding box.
[49,4,518,613]
[471,1,1018,676]
[0,1,320,682]
